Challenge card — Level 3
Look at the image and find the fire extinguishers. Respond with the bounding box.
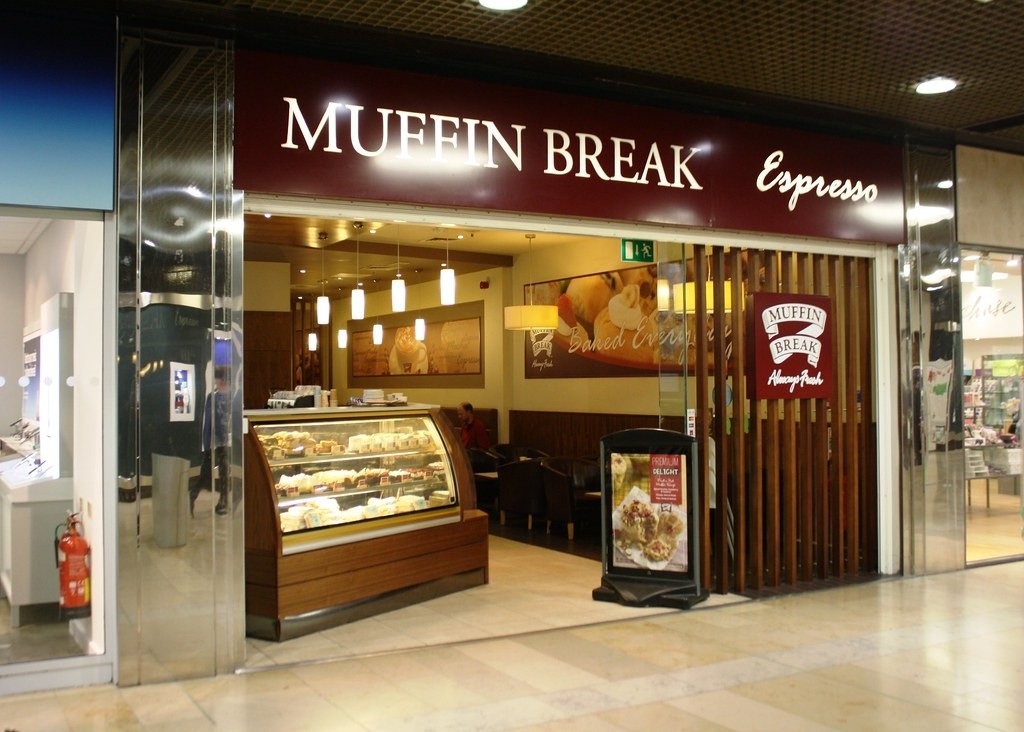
[53,512,91,609]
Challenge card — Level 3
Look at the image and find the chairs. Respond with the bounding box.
[467,444,602,541]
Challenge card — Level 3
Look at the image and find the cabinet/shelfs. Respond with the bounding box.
[962,374,1024,509]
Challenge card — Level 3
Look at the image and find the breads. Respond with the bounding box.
[255,430,453,533]
[553,264,730,368]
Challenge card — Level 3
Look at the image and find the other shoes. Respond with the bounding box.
[215,492,227,514]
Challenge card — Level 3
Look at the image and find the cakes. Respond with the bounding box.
[620,500,683,562]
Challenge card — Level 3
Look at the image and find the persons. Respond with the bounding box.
[189,365,235,521]
[456,401,492,454]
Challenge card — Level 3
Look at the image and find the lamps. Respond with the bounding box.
[316,232,330,325]
[439,238,455,306]
[414,269,425,341]
[674,244,745,315]
[351,221,365,320]
[372,278,384,344]
[504,233,558,331]
[338,288,347,349]
[656,275,669,311]
[391,224,406,312]
[308,294,318,351]
